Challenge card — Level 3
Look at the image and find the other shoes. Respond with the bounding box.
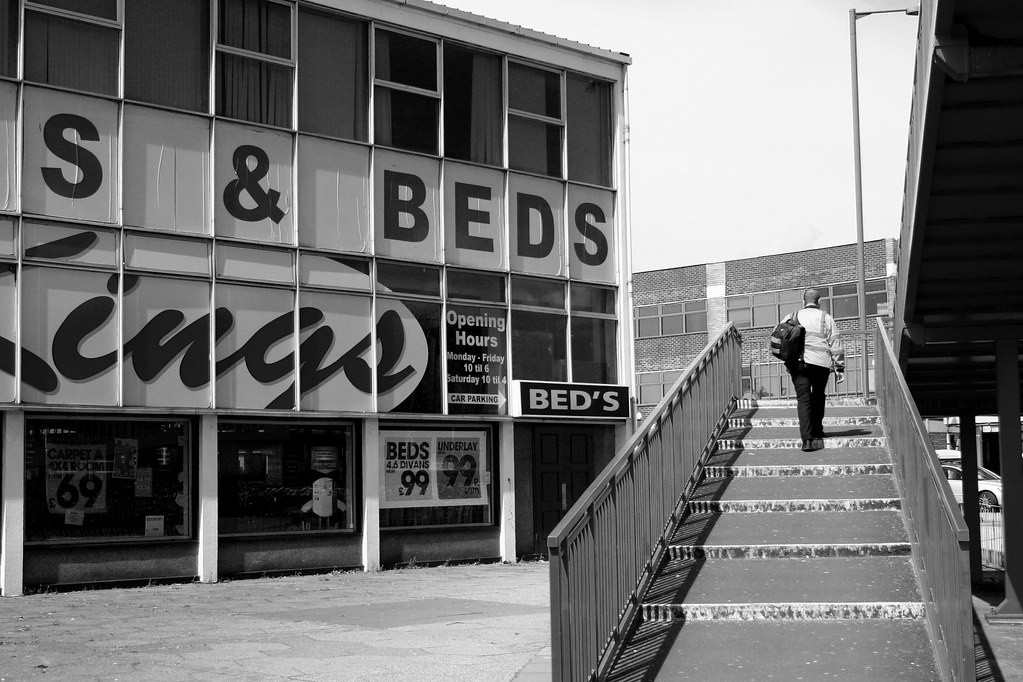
[802,439,813,451]
[810,430,826,439]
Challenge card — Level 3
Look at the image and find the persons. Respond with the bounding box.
[772,290,845,451]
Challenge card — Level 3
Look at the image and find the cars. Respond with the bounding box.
[940,463,1002,514]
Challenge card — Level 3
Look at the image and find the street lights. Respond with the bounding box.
[849,5,924,401]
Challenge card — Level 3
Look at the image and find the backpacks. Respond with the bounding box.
[769,311,805,361]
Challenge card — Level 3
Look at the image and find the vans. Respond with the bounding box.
[934,449,962,476]
